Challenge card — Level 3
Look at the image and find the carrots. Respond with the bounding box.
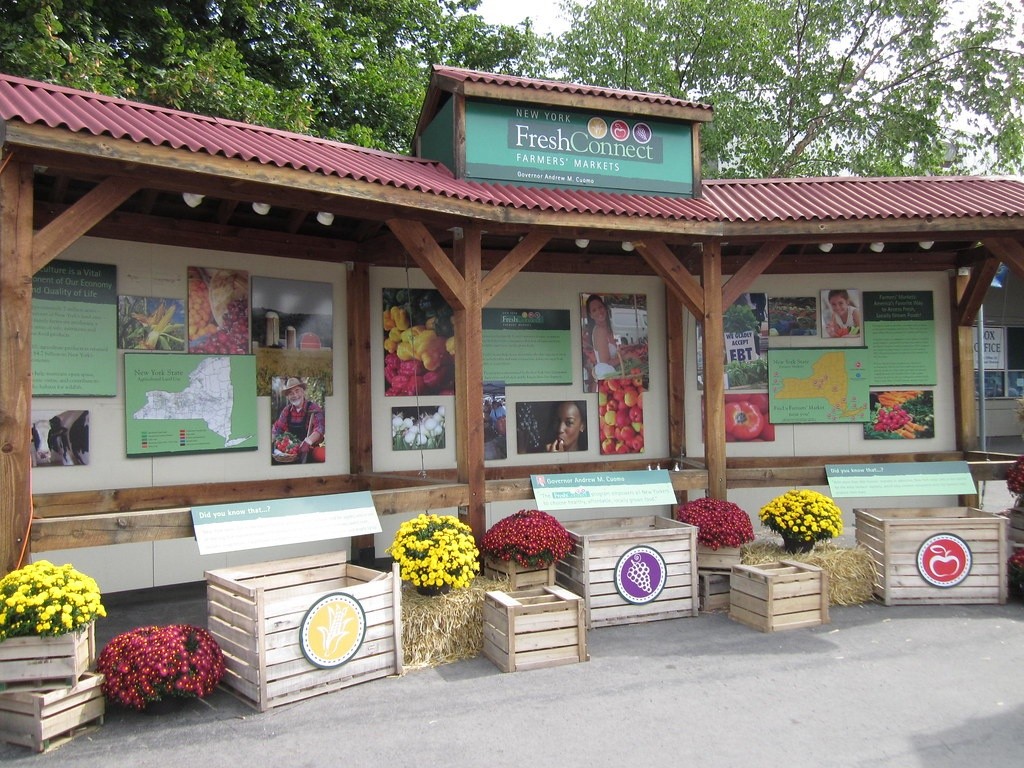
[896,421,922,439]
[878,391,920,409]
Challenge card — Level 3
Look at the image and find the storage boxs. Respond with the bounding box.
[854,506,1009,607]
[695,539,741,570]
[204,549,402,711]
[0,620,95,694]
[0,672,106,752]
[556,516,699,629]
[698,572,730,612]
[1007,506,1024,542]
[728,561,830,633]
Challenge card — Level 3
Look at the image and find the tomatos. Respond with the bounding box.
[725,393,775,442]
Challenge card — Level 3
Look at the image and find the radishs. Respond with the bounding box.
[276,435,300,454]
[873,404,910,432]
[189,298,248,355]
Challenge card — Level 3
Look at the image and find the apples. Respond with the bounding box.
[599,377,644,454]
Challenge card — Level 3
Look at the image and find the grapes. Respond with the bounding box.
[516,402,561,448]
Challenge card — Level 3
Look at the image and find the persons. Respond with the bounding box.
[542,402,588,453]
[490,402,506,424]
[586,294,635,392]
[827,289,860,337]
[271,377,324,462]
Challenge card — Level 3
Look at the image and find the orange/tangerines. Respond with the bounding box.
[188,270,217,341]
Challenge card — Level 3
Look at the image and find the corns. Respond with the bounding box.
[129,303,183,350]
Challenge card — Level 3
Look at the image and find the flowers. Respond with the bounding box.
[1006,455,1024,507]
[757,489,844,552]
[97,624,228,711]
[483,508,576,570]
[675,497,755,552]
[0,560,107,642]
[384,513,480,596]
[1009,547,1024,570]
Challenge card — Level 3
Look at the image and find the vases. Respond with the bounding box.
[143,698,180,715]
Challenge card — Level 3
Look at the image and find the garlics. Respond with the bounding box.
[391,407,444,450]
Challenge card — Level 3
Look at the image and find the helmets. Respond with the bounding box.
[282,377,307,396]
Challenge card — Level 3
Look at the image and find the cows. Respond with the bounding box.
[31,410,90,466]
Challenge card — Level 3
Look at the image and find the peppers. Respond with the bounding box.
[383,288,454,396]
